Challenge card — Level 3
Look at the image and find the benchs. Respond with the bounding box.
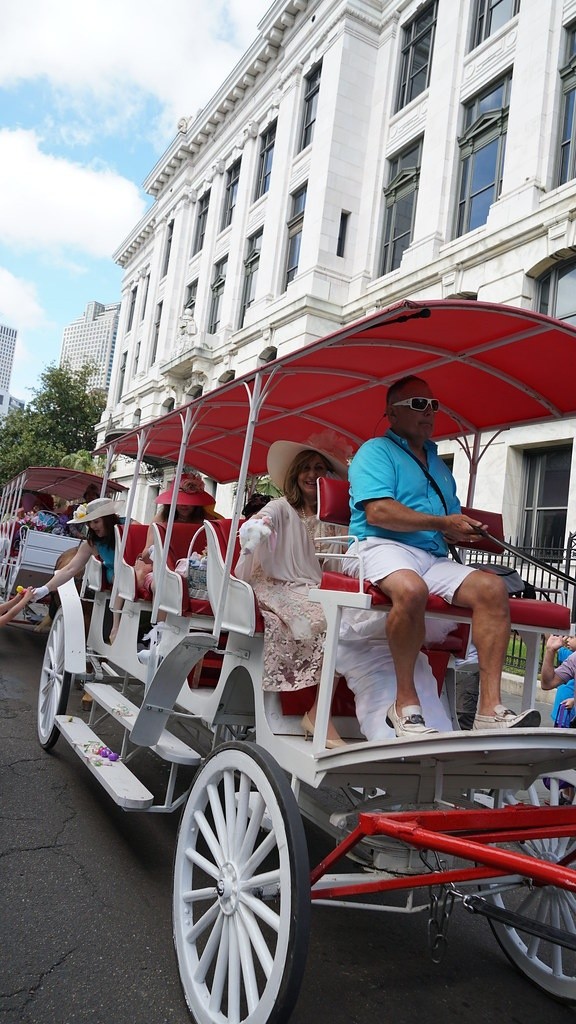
[0,519,70,581]
[202,517,473,736]
[302,474,573,755]
[84,522,153,657]
[152,518,230,693]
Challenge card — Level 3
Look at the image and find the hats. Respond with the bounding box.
[66,497,126,525]
[154,473,216,506]
[22,492,55,514]
[83,483,100,499]
[242,493,274,516]
[266,429,353,495]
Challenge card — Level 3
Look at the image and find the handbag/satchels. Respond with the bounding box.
[543,704,574,789]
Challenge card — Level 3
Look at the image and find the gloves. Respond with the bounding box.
[29,585,49,603]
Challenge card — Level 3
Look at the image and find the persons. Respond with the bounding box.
[541,621,576,806]
[236,448,384,751]
[0,484,142,644]
[134,483,217,624]
[341,373,541,739]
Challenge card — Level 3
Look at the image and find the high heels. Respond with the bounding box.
[301,712,348,749]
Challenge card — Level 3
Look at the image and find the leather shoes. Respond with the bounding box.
[471,704,541,729]
[386,702,438,737]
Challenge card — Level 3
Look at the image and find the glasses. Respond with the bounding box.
[393,397,439,412]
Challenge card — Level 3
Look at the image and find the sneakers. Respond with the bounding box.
[546,791,571,806]
